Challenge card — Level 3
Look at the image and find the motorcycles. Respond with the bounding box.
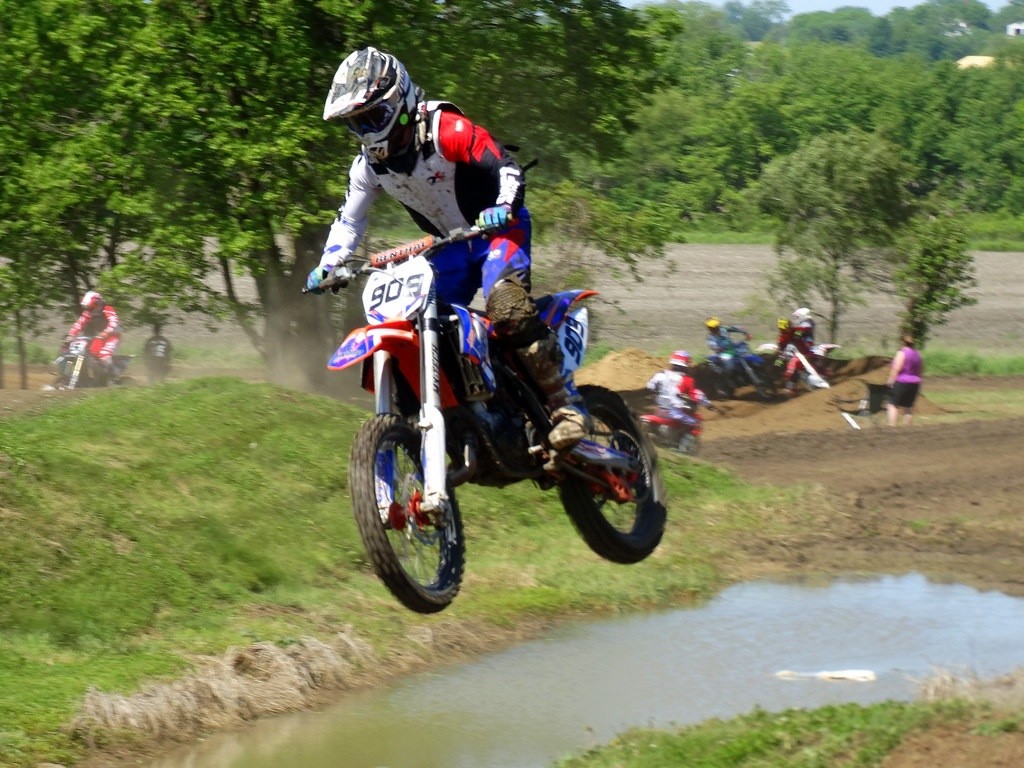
[300,210,667,615]
[634,388,726,456]
[41,336,136,390]
[757,343,842,394]
[711,338,778,402]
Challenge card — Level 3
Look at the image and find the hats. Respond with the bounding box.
[792,307,813,320]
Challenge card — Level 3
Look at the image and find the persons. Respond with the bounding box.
[646,349,714,452]
[703,308,816,398]
[304,45,592,450]
[886,337,923,428]
[58,291,123,383]
[145,326,171,377]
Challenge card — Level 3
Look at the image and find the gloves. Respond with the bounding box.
[479,203,513,240]
[306,265,339,294]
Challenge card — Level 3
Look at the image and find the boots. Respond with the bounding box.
[516,333,592,445]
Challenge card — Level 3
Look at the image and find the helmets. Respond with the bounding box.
[322,47,417,160]
[777,317,791,332]
[670,350,691,368]
[80,290,104,313]
[705,316,721,329]
